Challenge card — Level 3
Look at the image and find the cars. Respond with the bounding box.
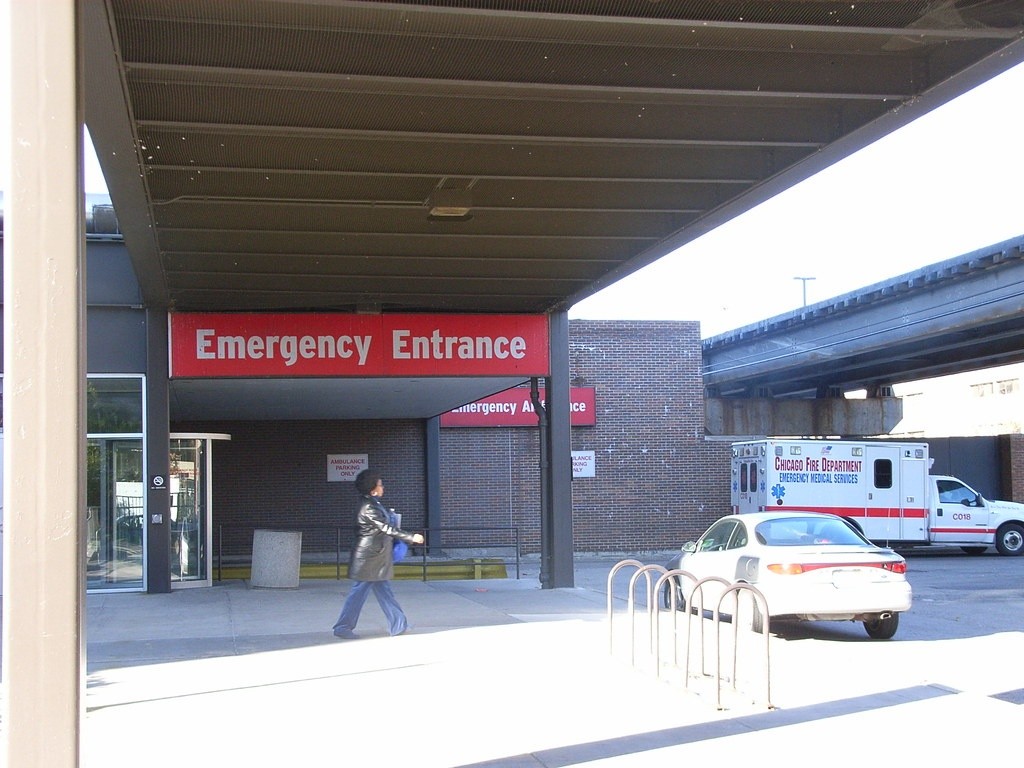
[116,515,195,549]
[662,510,911,640]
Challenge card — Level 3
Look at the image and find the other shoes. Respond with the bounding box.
[334,630,361,639]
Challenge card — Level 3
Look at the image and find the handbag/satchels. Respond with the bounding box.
[393,538,408,565]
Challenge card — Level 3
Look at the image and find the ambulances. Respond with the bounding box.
[731,439,1024,557]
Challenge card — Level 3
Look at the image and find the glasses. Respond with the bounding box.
[376,484,385,487]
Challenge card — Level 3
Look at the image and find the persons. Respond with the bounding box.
[332,468,425,639]
